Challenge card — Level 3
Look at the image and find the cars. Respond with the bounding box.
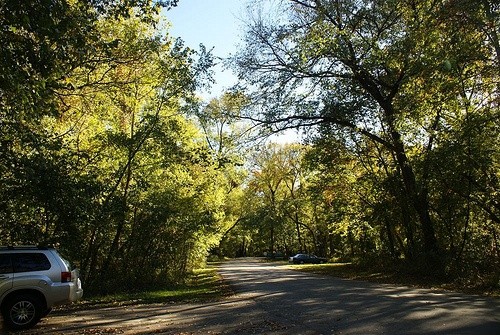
[289,254,328,264]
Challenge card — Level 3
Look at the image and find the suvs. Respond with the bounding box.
[0,243,83,328]
